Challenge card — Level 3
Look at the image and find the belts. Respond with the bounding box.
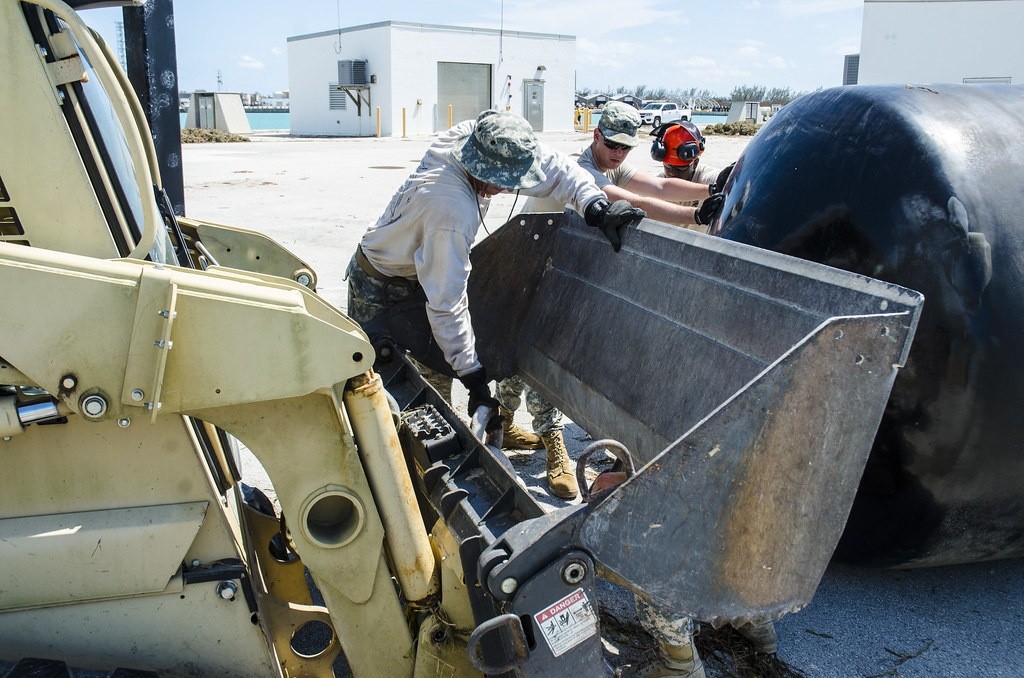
[355,243,415,287]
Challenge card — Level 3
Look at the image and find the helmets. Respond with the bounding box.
[656,119,705,167]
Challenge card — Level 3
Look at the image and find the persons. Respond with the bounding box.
[341,109,647,435]
[648,120,723,234]
[484,100,740,501]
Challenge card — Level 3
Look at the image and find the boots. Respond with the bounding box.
[539,431,578,499]
[485,402,544,450]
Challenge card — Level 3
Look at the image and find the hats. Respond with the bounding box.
[598,100,640,146]
[461,110,546,190]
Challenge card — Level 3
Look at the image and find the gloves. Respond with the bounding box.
[708,161,737,195]
[460,371,505,430]
[585,198,647,251]
[694,194,725,226]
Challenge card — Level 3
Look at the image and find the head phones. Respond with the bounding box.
[650,137,705,161]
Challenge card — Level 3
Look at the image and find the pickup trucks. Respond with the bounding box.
[637,104,692,127]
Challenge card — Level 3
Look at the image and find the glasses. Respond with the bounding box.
[598,130,632,151]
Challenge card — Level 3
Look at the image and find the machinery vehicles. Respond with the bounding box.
[0,0,923,678]
